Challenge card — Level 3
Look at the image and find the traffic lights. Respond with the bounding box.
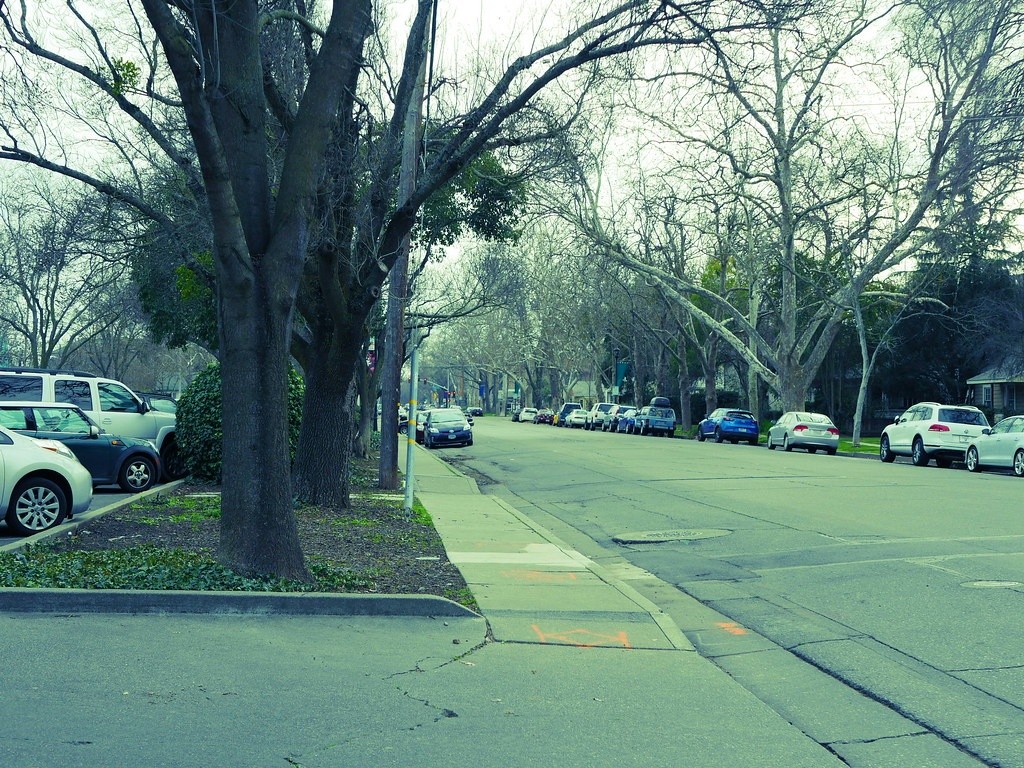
[453,391,456,398]
[443,391,448,399]
[408,377,411,384]
[418,375,421,383]
[448,392,451,398]
[424,378,427,385]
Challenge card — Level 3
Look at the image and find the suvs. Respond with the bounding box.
[601,406,638,433]
[879,402,993,470]
[633,397,677,439]
[100,389,180,415]
[584,403,619,431]
[557,403,583,428]
[0,367,184,483]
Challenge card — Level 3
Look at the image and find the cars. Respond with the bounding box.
[511,407,559,426]
[616,410,640,434]
[766,412,839,456]
[565,409,588,429]
[422,409,474,449]
[696,407,760,446]
[0,401,162,495]
[396,400,483,444]
[0,426,94,538]
[961,414,1024,477]
